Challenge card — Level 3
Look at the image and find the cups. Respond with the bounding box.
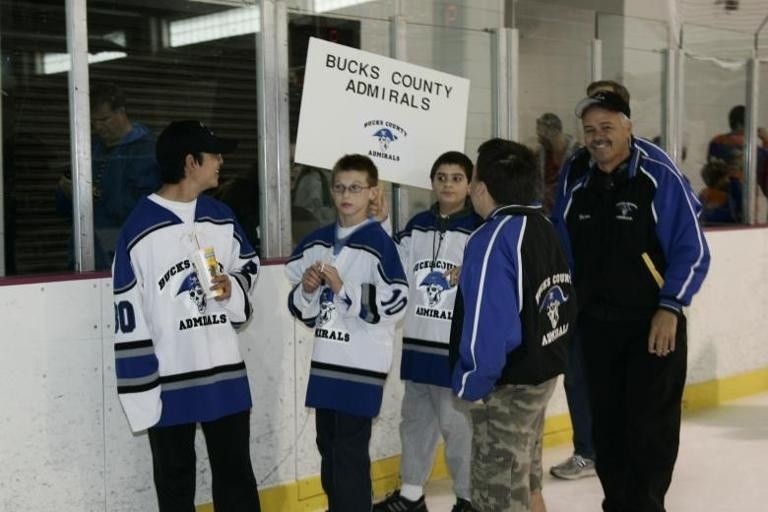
[187,244,225,302]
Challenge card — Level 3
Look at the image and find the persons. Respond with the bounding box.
[449,136,578,512]
[111,119,263,512]
[549,77,706,479]
[547,95,714,511]
[363,153,496,512]
[710,101,767,208]
[53,86,156,269]
[285,153,411,512]
[696,159,731,229]
[282,127,333,221]
[530,112,578,211]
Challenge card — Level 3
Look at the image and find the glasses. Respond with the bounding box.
[332,185,368,193]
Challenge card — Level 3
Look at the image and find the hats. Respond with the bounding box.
[575,90,630,119]
[158,119,238,164]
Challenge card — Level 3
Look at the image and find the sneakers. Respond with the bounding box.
[373,490,428,512]
[550,454,597,479]
[451,498,473,512]
